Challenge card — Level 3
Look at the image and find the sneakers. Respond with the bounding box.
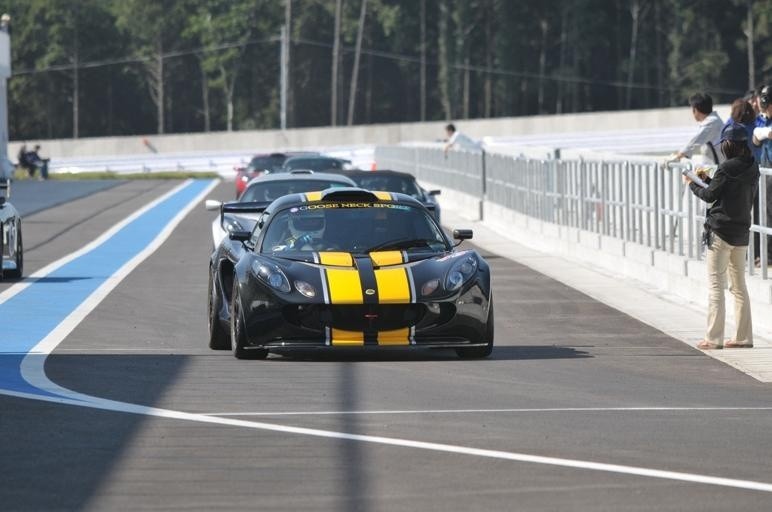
[696,340,755,349]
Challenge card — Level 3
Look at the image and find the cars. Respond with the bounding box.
[0,177,24,282]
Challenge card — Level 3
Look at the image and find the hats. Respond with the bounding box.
[713,122,748,146]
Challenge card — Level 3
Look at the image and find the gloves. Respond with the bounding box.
[294,234,312,246]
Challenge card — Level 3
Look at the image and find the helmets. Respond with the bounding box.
[288,213,327,240]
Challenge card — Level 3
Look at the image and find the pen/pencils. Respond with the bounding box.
[696,167,713,176]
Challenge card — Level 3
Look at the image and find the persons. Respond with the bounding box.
[660,84,771,350]
[443,124,484,154]
[19,145,50,180]
[272,207,337,251]
